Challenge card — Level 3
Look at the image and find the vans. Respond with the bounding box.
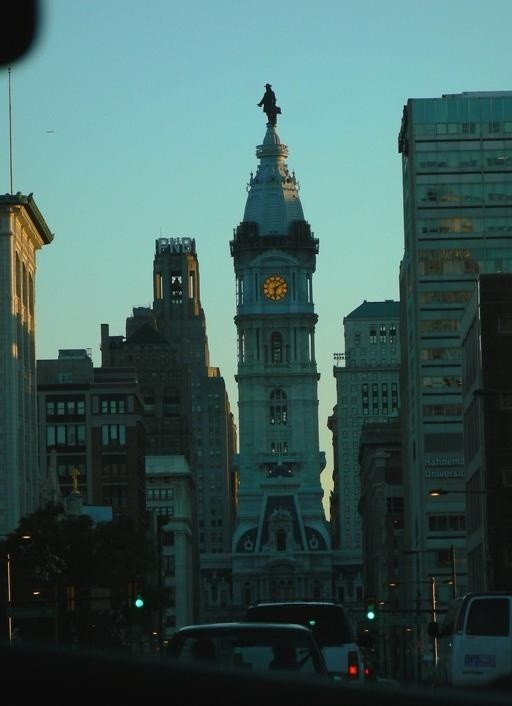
[429,590,512,695]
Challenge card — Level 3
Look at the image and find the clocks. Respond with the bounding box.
[264,274,288,302]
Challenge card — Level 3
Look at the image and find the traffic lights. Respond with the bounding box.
[133,584,146,609]
[364,594,376,622]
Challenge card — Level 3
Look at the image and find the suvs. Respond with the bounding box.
[243,599,367,685]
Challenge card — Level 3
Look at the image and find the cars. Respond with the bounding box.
[170,622,332,683]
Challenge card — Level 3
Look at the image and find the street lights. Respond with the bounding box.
[425,483,512,591]
[362,534,458,685]
